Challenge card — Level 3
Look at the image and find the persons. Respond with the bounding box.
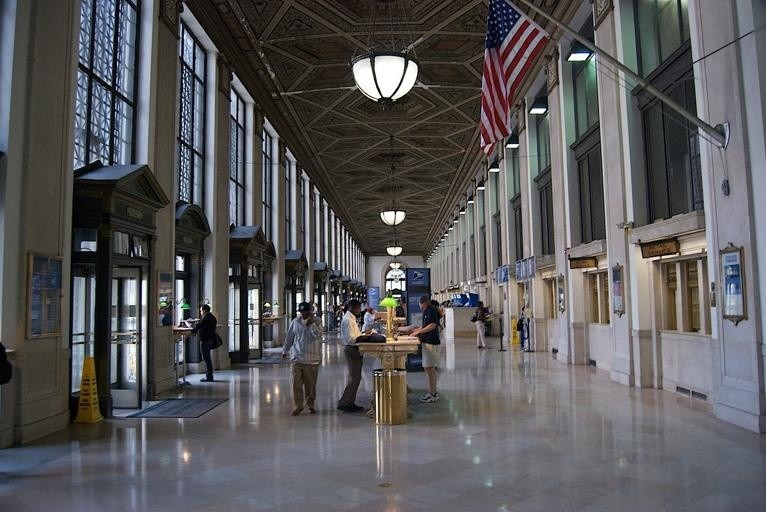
[520,305,525,348]
[282,302,323,416]
[474,301,490,349]
[0,342,12,384]
[195,304,217,382]
[313,303,322,317]
[327,296,454,413]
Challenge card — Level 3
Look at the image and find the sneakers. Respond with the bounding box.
[306,404,314,414]
[418,391,440,404]
[291,407,303,417]
[337,402,364,414]
[199,376,214,382]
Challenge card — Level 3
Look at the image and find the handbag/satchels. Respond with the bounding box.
[210,332,223,350]
[470,311,479,323]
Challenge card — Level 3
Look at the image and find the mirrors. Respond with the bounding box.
[156,269,174,328]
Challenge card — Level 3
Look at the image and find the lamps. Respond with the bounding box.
[347,0,423,109]
[387,226,404,260]
[378,292,401,341]
[173,297,193,310]
[389,256,401,271]
[272,299,281,308]
[159,301,167,308]
[424,42,594,263]
[379,163,408,232]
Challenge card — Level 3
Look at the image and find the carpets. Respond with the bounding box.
[123,398,229,418]
[247,356,296,365]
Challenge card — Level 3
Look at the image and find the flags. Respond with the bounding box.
[480,0,551,157]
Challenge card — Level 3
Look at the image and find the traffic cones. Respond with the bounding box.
[72,357,107,424]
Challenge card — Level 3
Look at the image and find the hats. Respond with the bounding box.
[297,302,312,313]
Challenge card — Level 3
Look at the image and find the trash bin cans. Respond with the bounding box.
[372,368,407,425]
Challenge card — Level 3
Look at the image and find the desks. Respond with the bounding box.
[262,316,283,326]
[354,316,422,419]
[172,327,195,386]
